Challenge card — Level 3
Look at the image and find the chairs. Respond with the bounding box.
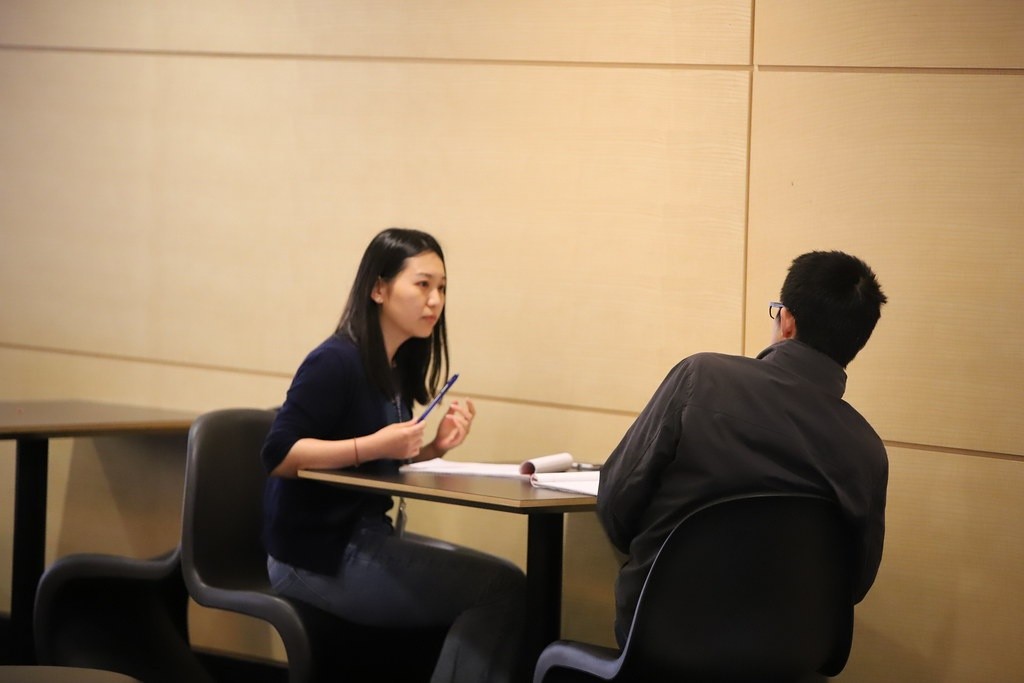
[184,406,526,681]
[530,491,853,682]
[34,516,186,683]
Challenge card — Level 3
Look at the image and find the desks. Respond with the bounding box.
[2,395,195,667]
[291,456,601,681]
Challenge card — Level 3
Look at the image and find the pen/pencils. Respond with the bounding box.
[415,373,459,425]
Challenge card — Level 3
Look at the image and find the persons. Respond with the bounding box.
[596,250,889,650]
[264,228,526,683]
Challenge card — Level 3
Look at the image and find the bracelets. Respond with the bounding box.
[354,438,359,467]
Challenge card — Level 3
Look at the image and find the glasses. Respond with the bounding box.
[768,302,789,319]
